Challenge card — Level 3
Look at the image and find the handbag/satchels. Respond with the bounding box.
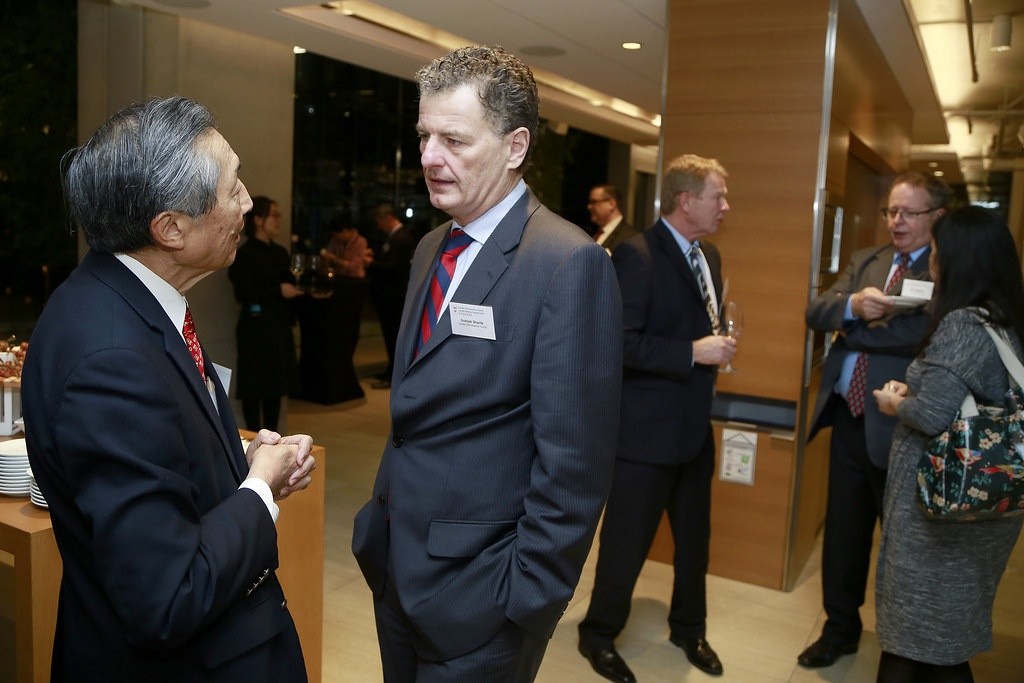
[915,306,1024,522]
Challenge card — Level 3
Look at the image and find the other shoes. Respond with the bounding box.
[372,379,390,389]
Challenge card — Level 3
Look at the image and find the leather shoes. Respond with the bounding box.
[668,631,723,674]
[578,640,636,683]
[798,638,857,668]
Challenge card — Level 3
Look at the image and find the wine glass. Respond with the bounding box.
[717,300,745,373]
[307,254,323,292]
[290,253,306,291]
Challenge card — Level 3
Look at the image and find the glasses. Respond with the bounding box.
[588,199,609,206]
[263,212,281,219]
[880,206,935,222]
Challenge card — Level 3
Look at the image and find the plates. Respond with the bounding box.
[0,438,49,508]
[885,295,927,309]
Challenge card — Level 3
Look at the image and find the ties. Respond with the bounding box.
[690,248,715,335]
[845,253,911,419]
[183,306,206,384]
[594,230,602,243]
[412,228,475,360]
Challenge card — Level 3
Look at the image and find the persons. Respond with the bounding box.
[226,193,312,436]
[872,202,1024,683]
[364,203,409,390]
[577,152,736,683]
[348,42,620,683]
[792,171,947,670]
[19,96,319,682]
[585,182,640,264]
[322,217,372,361]
